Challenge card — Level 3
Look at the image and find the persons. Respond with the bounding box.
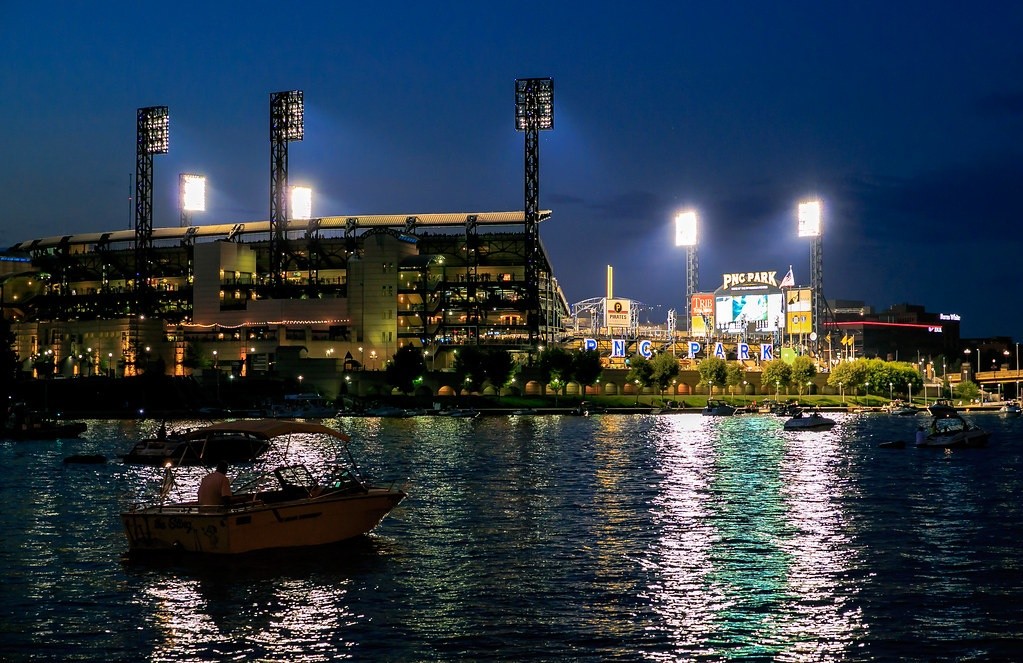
[916,427,929,445]
[198,462,245,513]
[813,411,817,418]
[930,420,937,434]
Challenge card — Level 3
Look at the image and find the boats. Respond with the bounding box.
[124,432,270,465]
[928,399,957,416]
[892,408,919,415]
[702,398,736,416]
[338,406,427,415]
[0,403,87,441]
[439,409,477,416]
[571,408,607,416]
[784,414,837,428]
[121,421,406,555]
[927,414,988,447]
[1006,403,1020,413]
[266,394,340,417]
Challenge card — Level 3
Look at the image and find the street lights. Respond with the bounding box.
[370,351,378,369]
[672,380,812,400]
[79,348,112,376]
[596,378,639,403]
[838,382,1001,406]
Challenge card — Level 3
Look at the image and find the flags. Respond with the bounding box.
[848,337,854,345]
[841,336,848,345]
[824,334,830,343]
